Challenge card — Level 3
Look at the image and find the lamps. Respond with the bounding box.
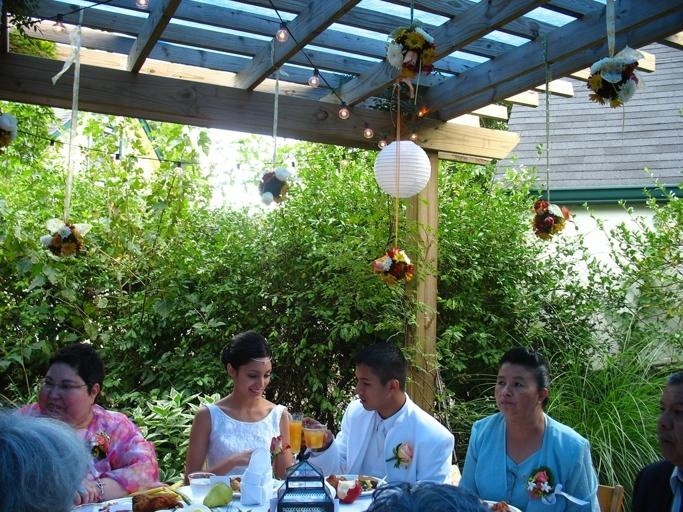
[270,447,335,512]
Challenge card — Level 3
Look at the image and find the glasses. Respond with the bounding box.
[41,378,90,395]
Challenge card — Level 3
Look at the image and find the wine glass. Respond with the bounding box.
[288,412,303,467]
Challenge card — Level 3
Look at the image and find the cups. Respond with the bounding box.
[301,423,326,451]
[188,473,216,501]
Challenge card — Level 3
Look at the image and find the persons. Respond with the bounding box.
[630,369,683,512]
[2,340,162,505]
[182,328,297,486]
[452,347,603,511]
[362,480,500,511]
[0,407,100,512]
[299,334,456,486]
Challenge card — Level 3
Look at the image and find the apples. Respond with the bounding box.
[335,479,362,504]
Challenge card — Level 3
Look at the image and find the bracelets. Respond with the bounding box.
[92,478,105,498]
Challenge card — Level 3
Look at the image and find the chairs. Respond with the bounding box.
[591,482,626,512]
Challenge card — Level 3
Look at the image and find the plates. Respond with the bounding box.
[480,498,522,511]
[325,474,387,497]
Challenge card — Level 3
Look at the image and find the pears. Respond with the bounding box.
[203,482,233,507]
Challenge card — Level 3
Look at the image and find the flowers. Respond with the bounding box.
[531,199,569,242]
[266,436,289,465]
[380,441,412,471]
[523,465,554,502]
[369,249,418,289]
[38,219,87,258]
[381,24,439,82]
[85,430,112,462]
[255,167,294,207]
[582,55,640,110]
